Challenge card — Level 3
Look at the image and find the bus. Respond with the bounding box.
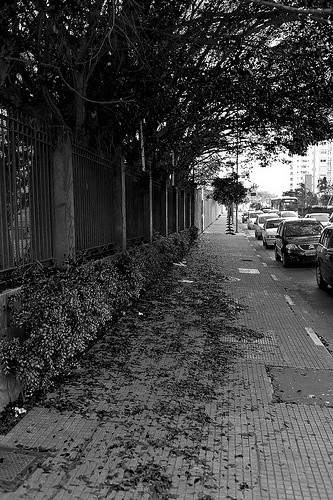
[271,196,302,218]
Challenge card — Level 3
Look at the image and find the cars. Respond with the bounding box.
[305,213,331,228]
[316,224,333,290]
[259,218,287,250]
[241,208,301,224]
[246,211,264,229]
[254,213,279,240]
[275,219,325,268]
